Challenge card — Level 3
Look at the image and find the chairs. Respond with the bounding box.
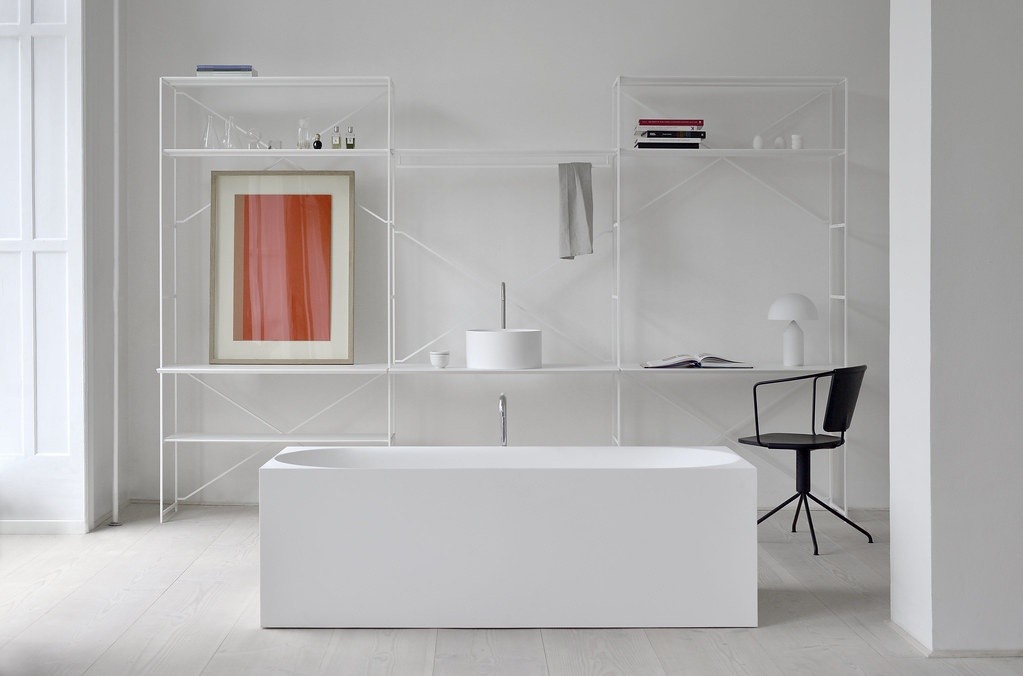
[738,364,875,556]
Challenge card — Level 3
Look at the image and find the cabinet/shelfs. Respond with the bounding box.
[158,76,849,524]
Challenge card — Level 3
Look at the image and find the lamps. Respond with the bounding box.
[769,295,818,367]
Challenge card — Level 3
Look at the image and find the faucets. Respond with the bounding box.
[496,392,508,447]
[500,281,507,329]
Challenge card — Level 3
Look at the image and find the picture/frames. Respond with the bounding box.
[210,170,355,366]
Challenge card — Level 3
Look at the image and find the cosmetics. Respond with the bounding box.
[297,118,356,148]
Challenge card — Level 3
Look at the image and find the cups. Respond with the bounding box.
[430,351,450,370]
[297,127,311,149]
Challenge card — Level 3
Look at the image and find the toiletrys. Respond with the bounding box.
[296,118,310,149]
[313,133,323,149]
[345,126,356,149]
[331,125,342,149]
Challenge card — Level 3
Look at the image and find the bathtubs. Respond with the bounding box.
[258,444,758,627]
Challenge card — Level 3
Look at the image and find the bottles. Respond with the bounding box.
[313,134,322,149]
[331,126,341,149]
[202,114,219,149]
[345,126,356,149]
[221,113,242,149]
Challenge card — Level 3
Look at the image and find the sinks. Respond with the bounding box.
[465,328,543,369]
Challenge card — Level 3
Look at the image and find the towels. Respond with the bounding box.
[557,162,594,261]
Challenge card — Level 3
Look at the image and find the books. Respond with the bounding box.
[639,352,754,369]
[195,64,258,77]
[633,117,707,149]
